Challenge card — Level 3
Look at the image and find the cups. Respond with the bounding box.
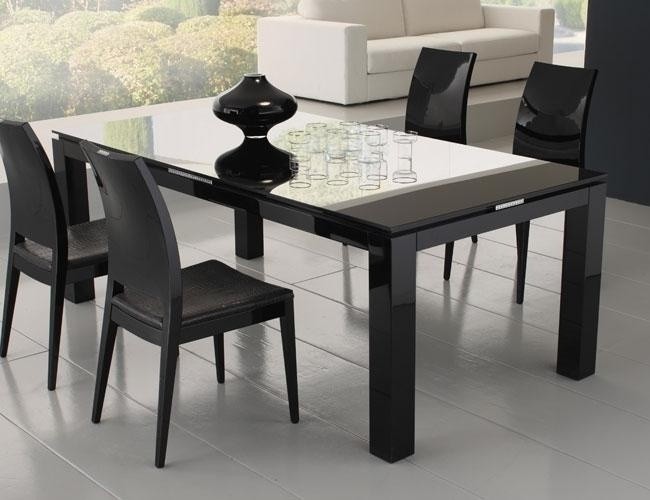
[288,120,418,162]
[290,150,420,190]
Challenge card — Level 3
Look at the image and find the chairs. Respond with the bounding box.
[511,47,597,305]
[340,45,477,280]
[1,118,119,391]
[67,138,302,468]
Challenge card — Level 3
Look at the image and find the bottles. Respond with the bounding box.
[215,137,299,192]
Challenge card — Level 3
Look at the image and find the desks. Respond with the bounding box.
[42,90,611,464]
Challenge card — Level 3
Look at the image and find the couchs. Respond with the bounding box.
[254,0,555,107]
